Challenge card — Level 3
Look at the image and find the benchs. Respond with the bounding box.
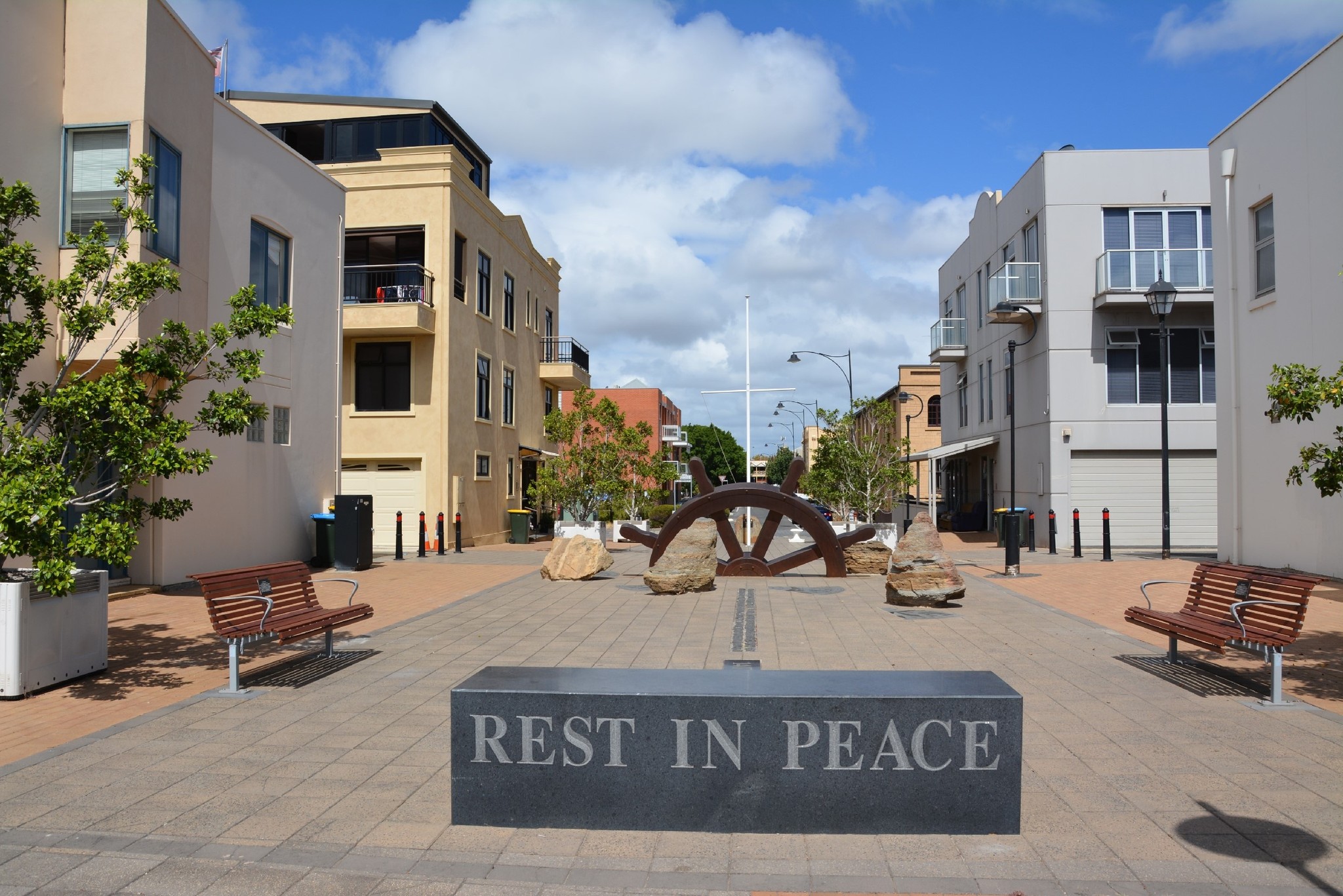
[180,562,376,699]
[1123,557,1333,706]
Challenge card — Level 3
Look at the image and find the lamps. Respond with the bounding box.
[1061,428,1072,437]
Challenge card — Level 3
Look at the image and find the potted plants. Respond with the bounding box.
[795,396,920,555]
[0,148,295,708]
[526,384,685,548]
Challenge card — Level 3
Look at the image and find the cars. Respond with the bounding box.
[791,493,833,528]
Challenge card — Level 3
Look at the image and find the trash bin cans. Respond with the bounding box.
[992,507,1028,546]
[311,513,336,567]
[507,509,533,544]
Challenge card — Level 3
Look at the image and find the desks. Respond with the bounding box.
[378,285,426,303]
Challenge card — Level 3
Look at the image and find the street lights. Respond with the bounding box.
[985,302,1039,574]
[776,400,820,467]
[768,422,795,459]
[763,442,784,456]
[1142,269,1180,558]
[786,349,855,449]
[892,391,924,536]
[772,408,807,473]
